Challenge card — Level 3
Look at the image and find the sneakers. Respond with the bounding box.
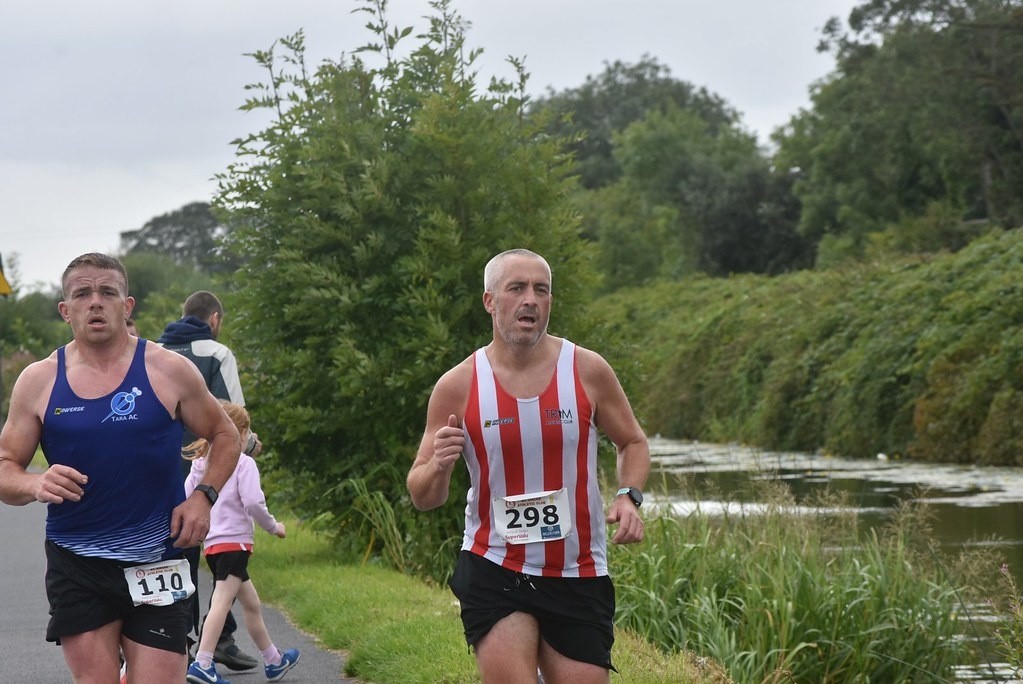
[186,661,230,684]
[264,648,300,681]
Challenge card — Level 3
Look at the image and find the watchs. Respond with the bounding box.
[194,484,219,505]
[616,486,643,507]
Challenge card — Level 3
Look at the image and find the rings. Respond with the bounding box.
[199,538,204,543]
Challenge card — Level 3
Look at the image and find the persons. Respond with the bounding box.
[407,249,650,684]
[0,252,241,684]
[181,398,302,684]
[126,319,138,337]
[155,290,262,671]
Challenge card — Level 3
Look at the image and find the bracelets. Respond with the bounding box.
[250,440,257,453]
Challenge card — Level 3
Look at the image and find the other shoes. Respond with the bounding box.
[120,656,127,684]
[213,640,260,666]
[214,658,258,676]
